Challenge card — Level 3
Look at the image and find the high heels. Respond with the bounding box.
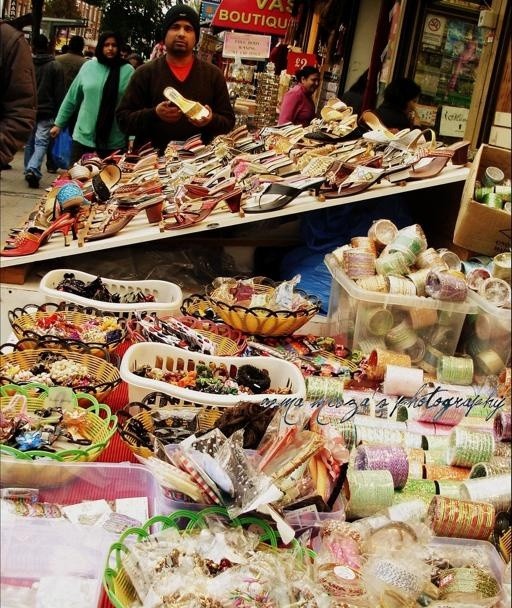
[4,111,470,257]
[163,87,210,120]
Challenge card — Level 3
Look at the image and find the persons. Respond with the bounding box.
[115,2,236,154]
[377,77,421,129]
[0,16,39,170]
[48,31,136,156]
[276,67,322,127]
[20,34,164,186]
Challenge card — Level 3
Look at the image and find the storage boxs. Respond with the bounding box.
[454,145,512,257]
[323,221,478,385]
[448,258,510,384]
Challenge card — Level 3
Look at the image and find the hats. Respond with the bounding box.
[163,5,199,44]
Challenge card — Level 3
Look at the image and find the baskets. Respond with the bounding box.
[4,271,365,607]
